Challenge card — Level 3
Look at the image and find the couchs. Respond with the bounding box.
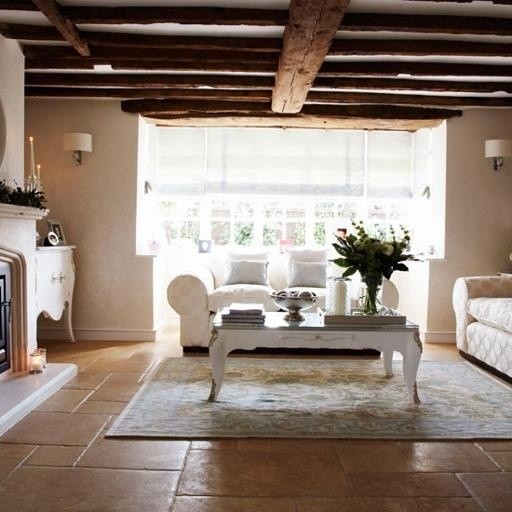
[455,272,512,387]
[170,246,400,349]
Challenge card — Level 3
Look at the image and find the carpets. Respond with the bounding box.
[103,357,512,443]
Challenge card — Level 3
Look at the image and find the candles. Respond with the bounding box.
[36,165,41,187]
[29,137,35,176]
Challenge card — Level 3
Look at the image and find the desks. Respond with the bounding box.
[34,246,79,344]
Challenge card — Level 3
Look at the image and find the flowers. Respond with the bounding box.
[331,219,435,313]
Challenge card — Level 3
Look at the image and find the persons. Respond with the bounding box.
[52,224,63,242]
[50,235,57,244]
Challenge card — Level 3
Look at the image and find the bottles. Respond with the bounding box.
[29,348,47,375]
[327,276,352,317]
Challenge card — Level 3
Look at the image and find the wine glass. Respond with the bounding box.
[270,292,318,322]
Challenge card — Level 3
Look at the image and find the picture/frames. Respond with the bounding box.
[47,220,67,245]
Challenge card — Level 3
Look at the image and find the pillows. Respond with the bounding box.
[221,247,328,288]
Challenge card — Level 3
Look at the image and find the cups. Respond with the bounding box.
[195,238,212,253]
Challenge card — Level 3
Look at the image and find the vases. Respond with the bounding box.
[362,286,379,313]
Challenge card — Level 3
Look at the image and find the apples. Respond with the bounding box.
[276,290,317,297]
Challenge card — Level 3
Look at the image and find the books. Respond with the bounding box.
[223,323,264,327]
[221,306,265,319]
[229,302,263,315]
[222,319,265,324]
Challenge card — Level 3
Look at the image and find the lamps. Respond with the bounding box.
[485,139,512,171]
[62,132,93,166]
